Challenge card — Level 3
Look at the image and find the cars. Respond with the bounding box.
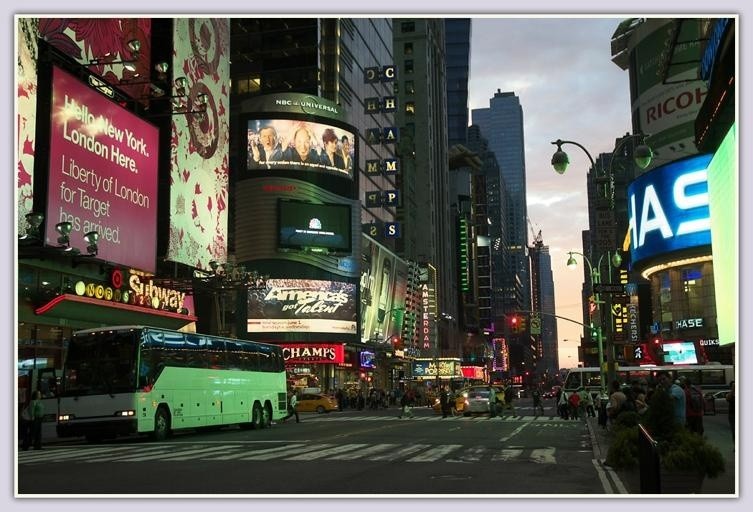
[432,383,562,418]
[707,390,731,411]
[294,391,340,415]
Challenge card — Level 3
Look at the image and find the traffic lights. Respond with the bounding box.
[512,317,518,335]
[632,346,642,360]
[519,317,525,335]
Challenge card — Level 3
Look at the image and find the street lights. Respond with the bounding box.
[544,132,657,400]
[565,248,625,396]
[373,307,406,383]
[431,311,454,384]
[563,338,580,344]
[565,354,577,359]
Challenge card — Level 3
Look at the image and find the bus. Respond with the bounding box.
[556,364,733,414]
[56,325,289,442]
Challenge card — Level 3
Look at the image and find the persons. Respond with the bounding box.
[283,391,302,424]
[596,372,706,453]
[28,390,46,450]
[248,123,353,176]
[725,381,734,441]
[531,387,595,421]
[328,385,418,419]
[439,381,518,419]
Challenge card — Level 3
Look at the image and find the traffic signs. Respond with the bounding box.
[592,282,623,294]
